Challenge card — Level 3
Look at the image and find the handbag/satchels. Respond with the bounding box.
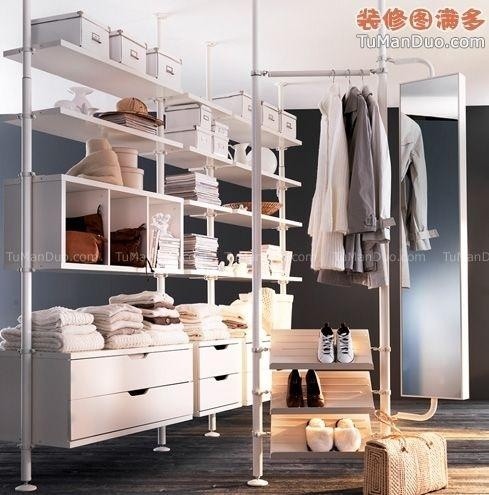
[112,222,144,267]
[66,215,107,264]
[362,431,449,495]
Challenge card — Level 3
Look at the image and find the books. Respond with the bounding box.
[165,170,222,206]
[99,113,159,137]
[149,228,220,271]
[234,245,293,277]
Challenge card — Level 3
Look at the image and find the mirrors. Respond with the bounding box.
[398,71,469,401]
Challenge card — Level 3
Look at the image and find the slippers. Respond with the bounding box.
[333,417,362,452]
[305,417,334,451]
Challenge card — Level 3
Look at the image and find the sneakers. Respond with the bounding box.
[318,321,336,364]
[336,323,354,363]
[305,369,324,407]
[287,369,302,406]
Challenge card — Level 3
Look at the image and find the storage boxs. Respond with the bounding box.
[163,102,230,158]
[208,88,298,138]
[28,10,183,88]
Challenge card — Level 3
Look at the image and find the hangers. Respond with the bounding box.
[317,68,383,111]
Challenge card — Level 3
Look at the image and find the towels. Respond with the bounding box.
[0,288,250,349]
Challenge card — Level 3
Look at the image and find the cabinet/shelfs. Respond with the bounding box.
[0,0,303,495]
[269,328,376,453]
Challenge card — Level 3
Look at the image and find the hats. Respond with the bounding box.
[94,97,164,126]
